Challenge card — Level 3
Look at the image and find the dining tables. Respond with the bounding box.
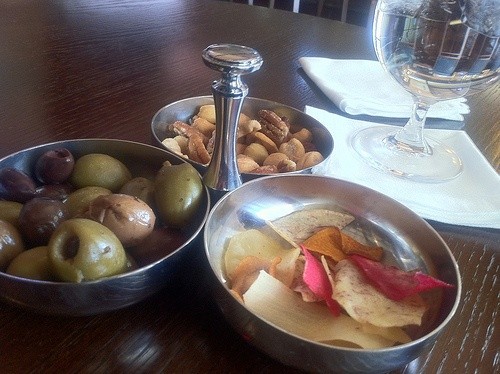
[0,0,499,373]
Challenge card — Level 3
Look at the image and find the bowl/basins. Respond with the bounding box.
[203,174,462,373]
[0,138,211,317]
[151,94,334,184]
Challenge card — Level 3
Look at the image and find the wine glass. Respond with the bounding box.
[351,0,500,183]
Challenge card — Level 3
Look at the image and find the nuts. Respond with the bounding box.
[162,102,325,173]
